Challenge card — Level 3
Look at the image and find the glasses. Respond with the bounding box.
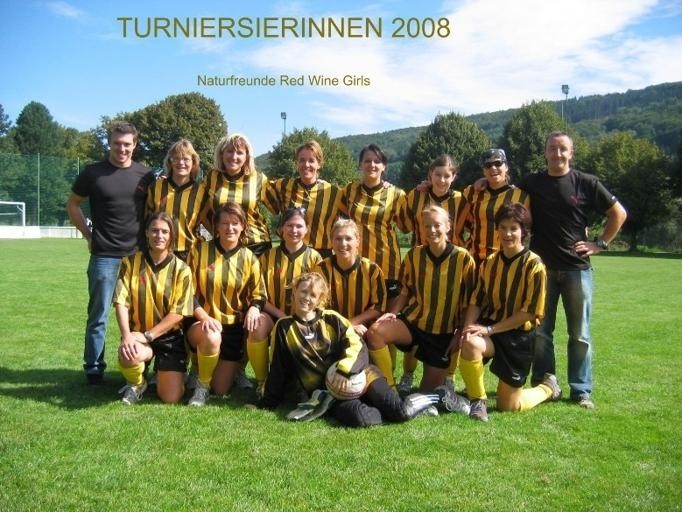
[484,160,505,168]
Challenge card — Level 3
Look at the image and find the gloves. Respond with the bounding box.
[301,394,337,422]
[287,389,327,420]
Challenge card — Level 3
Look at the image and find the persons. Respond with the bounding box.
[202,131,281,394]
[116,138,211,391]
[343,143,414,388]
[110,209,194,406]
[245,203,325,405]
[396,151,488,397]
[461,145,532,398]
[186,199,269,409]
[66,121,156,387]
[517,128,630,410]
[309,216,388,338]
[460,200,564,413]
[258,140,350,260]
[246,271,472,428]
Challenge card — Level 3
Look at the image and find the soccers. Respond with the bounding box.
[326,360,366,400]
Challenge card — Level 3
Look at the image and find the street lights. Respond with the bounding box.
[561,82,570,119]
[280,111,287,135]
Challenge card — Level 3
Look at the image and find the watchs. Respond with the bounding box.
[144,329,153,343]
[596,238,609,250]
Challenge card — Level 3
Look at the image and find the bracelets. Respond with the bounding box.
[487,325,496,337]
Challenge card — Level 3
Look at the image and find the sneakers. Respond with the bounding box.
[88,369,253,409]
[576,397,594,409]
[398,373,413,393]
[426,405,438,416]
[469,398,488,422]
[543,372,562,401]
[433,385,471,416]
